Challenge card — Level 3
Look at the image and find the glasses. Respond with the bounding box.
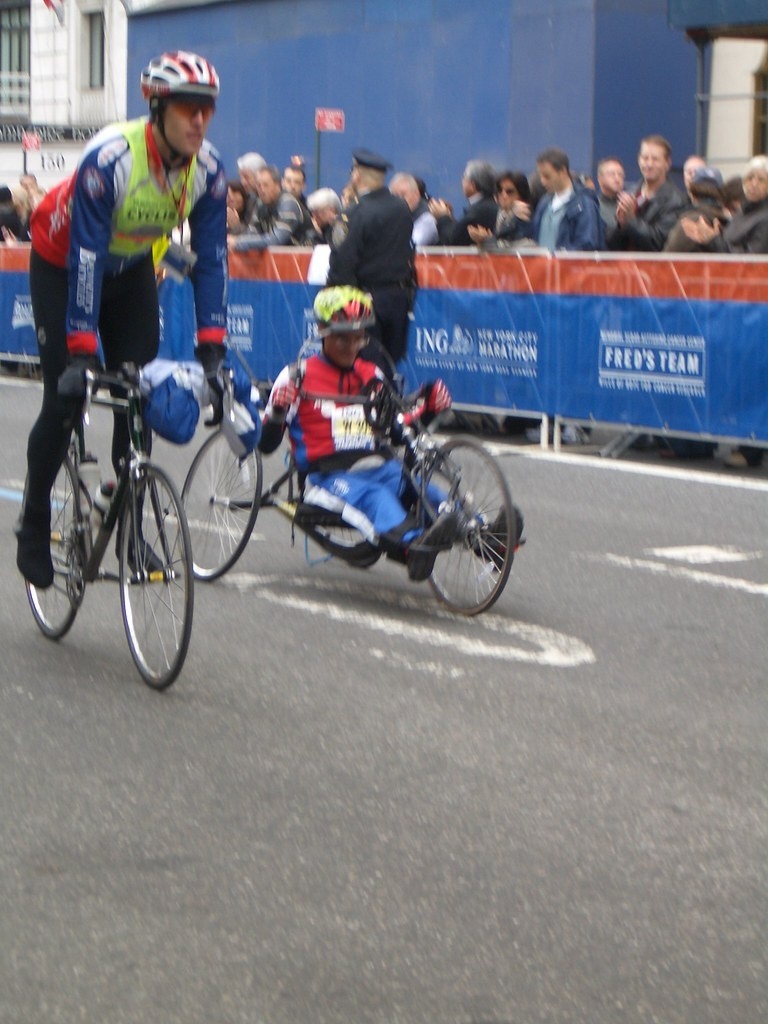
[323,327,369,355]
[162,88,217,122]
[495,184,518,196]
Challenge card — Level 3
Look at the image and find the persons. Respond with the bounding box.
[258,286,523,582]
[388,136,768,467]
[13,50,226,590]
[225,153,357,406]
[0,174,47,378]
[328,148,416,375]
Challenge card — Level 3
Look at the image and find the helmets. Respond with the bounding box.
[139,50,221,100]
[313,283,378,331]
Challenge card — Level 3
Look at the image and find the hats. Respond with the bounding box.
[348,146,396,174]
[690,165,724,190]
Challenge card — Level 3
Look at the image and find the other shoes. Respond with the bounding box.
[404,511,449,583]
[13,516,54,590]
[473,506,524,573]
[116,530,165,580]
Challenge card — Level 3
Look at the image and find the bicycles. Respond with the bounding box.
[23,333,194,692]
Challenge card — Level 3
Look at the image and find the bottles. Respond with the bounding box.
[90,481,115,527]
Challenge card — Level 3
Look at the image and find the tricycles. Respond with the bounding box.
[179,373,517,618]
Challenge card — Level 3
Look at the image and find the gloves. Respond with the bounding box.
[194,343,233,398]
[403,379,452,436]
[268,384,300,424]
[55,352,107,403]
